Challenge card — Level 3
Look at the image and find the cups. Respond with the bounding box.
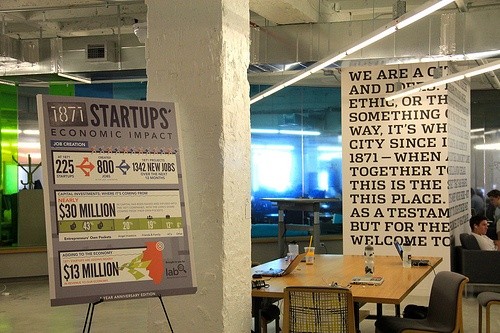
[304,247,315,264]
[287,253,297,272]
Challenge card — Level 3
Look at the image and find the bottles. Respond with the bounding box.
[288,240,298,256]
[403,243,411,267]
[364,245,375,274]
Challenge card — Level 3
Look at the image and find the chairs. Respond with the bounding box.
[281,286,369,333]
[374,232,500,333]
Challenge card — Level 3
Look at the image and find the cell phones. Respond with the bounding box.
[350,275,385,285]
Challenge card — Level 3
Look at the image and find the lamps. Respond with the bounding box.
[248,0,500,105]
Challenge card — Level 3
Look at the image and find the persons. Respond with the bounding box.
[487,189,500,240]
[469,214,496,251]
[470,187,484,216]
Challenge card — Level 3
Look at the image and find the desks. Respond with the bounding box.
[259,197,342,257]
[251,252,443,333]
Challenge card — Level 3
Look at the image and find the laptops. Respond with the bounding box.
[395,239,432,266]
[253,251,308,277]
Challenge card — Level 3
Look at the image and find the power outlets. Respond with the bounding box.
[321,243,324,248]
